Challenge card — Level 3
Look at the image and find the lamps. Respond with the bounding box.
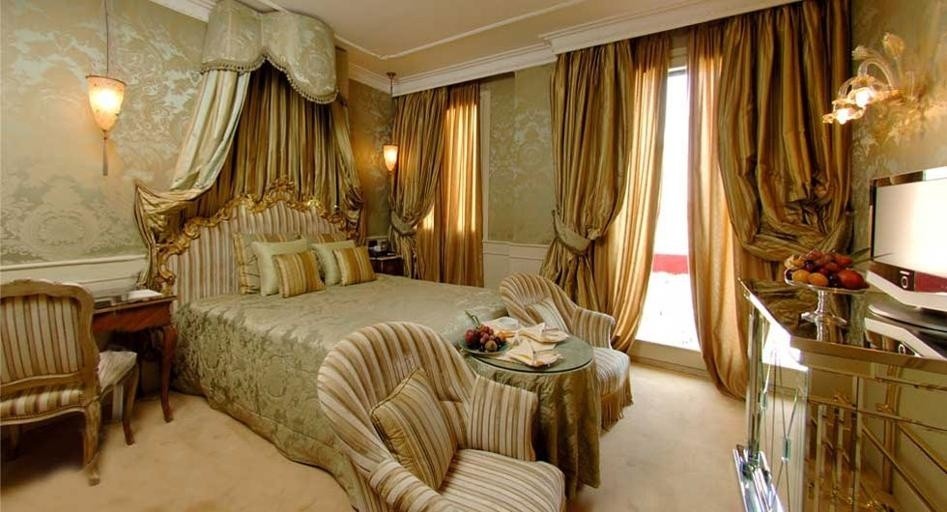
[383,70,399,172]
[822,30,927,125]
[84,0,128,175]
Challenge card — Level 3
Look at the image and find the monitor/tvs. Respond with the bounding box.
[865,166,946,333]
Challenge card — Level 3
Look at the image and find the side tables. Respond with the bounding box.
[457,326,601,499]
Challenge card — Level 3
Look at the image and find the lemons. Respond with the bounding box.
[484,340,498,352]
[498,332,507,342]
[792,269,829,287]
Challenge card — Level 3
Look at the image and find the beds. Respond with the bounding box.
[153,177,507,512]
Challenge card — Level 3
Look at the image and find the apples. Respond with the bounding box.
[838,270,864,290]
[806,249,853,271]
[465,330,480,350]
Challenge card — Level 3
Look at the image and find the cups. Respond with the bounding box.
[496,317,519,329]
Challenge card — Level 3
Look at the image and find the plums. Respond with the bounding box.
[802,261,839,288]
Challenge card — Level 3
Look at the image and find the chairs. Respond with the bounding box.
[315,320,569,512]
[499,269,633,431]
[1,277,139,485]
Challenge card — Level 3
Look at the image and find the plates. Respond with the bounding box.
[487,318,526,333]
[457,336,511,356]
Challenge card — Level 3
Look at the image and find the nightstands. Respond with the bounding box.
[90,288,177,422]
[369,254,405,277]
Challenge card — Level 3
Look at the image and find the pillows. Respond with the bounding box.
[307,230,348,281]
[250,235,306,295]
[368,366,457,491]
[232,229,299,296]
[310,239,358,288]
[271,248,326,297]
[525,296,571,333]
[332,245,376,285]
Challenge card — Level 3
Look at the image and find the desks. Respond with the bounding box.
[731,275,947,512]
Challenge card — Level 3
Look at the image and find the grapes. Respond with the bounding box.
[474,323,501,353]
